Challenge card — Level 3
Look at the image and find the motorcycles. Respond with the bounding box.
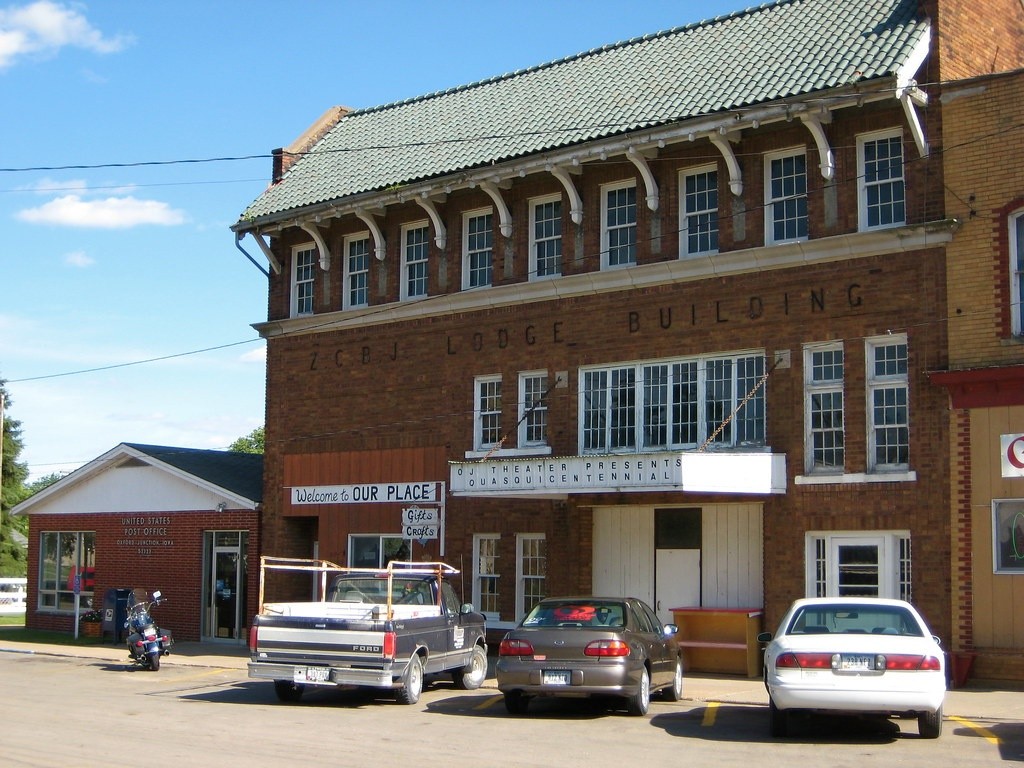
[124,588,170,671]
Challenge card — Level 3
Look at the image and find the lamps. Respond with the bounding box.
[215,503,227,512]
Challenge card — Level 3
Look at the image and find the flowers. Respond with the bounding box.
[79,609,102,623]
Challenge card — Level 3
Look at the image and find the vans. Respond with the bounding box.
[68,566,95,607]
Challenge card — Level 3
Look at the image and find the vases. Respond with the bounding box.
[83,622,102,637]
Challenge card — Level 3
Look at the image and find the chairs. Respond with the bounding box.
[344,590,364,603]
[609,617,623,628]
[407,592,423,604]
[801,625,899,634]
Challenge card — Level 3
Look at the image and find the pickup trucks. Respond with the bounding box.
[247,555,488,705]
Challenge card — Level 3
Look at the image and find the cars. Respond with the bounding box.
[495,597,683,716]
[757,597,946,739]
